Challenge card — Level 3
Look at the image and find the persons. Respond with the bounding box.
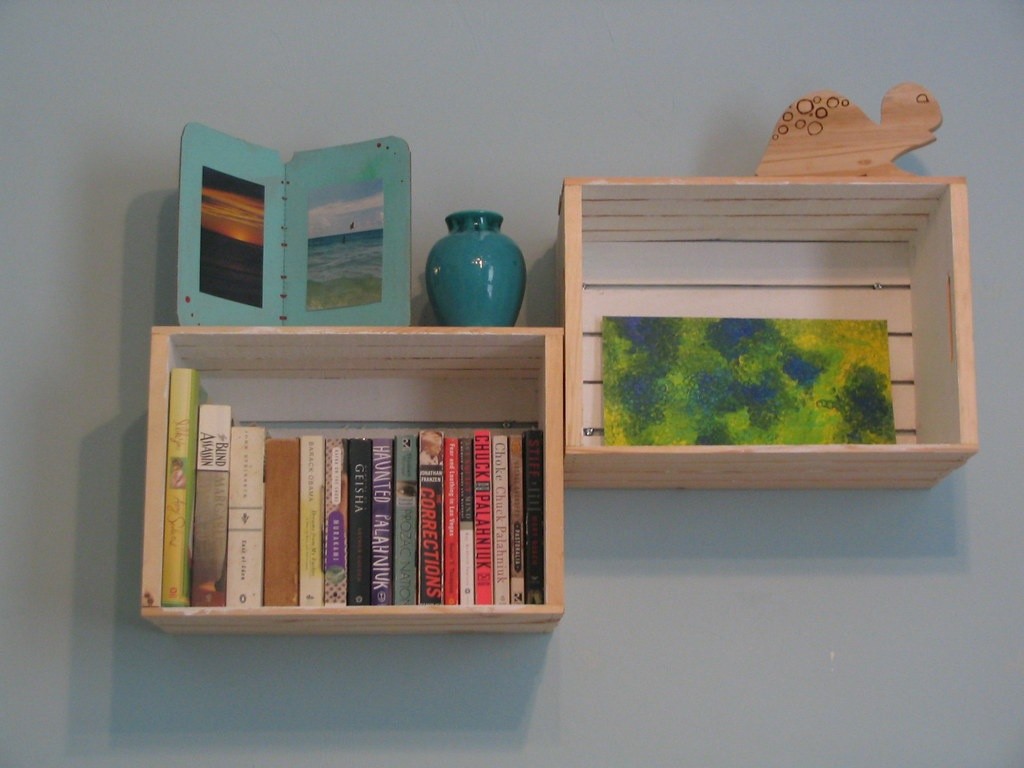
[419,432,444,465]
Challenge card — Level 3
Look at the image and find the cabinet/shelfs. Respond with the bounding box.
[141,326,567,633]
[558,174,980,491]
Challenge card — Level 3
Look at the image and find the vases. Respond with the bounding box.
[424,209,527,327]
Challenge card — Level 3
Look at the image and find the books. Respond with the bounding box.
[161,366,543,609]
